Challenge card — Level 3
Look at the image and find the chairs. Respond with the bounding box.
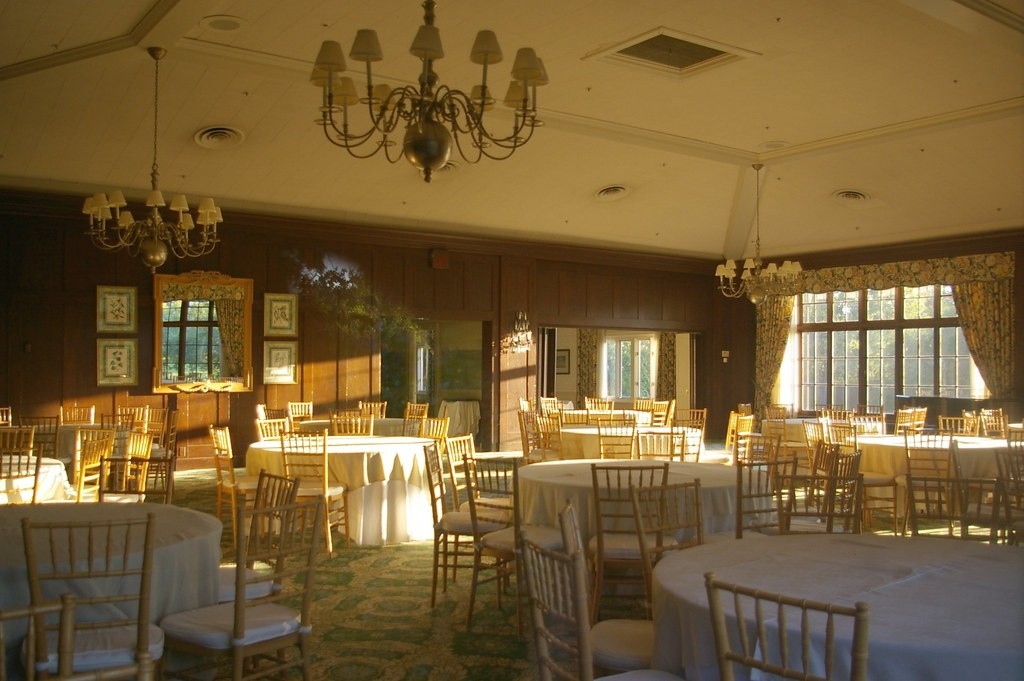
[0,396,1024,680]
[162,372,234,384]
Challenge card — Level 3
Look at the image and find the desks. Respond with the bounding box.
[650,534,1024,681]
[761,419,886,508]
[300,418,433,439]
[0,424,136,485]
[1006,423,1024,440]
[847,435,1024,538]
[550,410,654,429]
[0,502,224,681]
[510,459,773,544]
[0,456,78,504]
[550,427,705,463]
[243,435,446,546]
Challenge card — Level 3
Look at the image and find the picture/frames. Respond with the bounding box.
[96,285,138,333]
[96,338,139,386]
[263,293,299,337]
[556,349,570,374]
[263,341,299,385]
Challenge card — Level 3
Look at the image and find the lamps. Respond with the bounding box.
[309,0,549,182]
[82,47,224,274]
[715,164,802,305]
